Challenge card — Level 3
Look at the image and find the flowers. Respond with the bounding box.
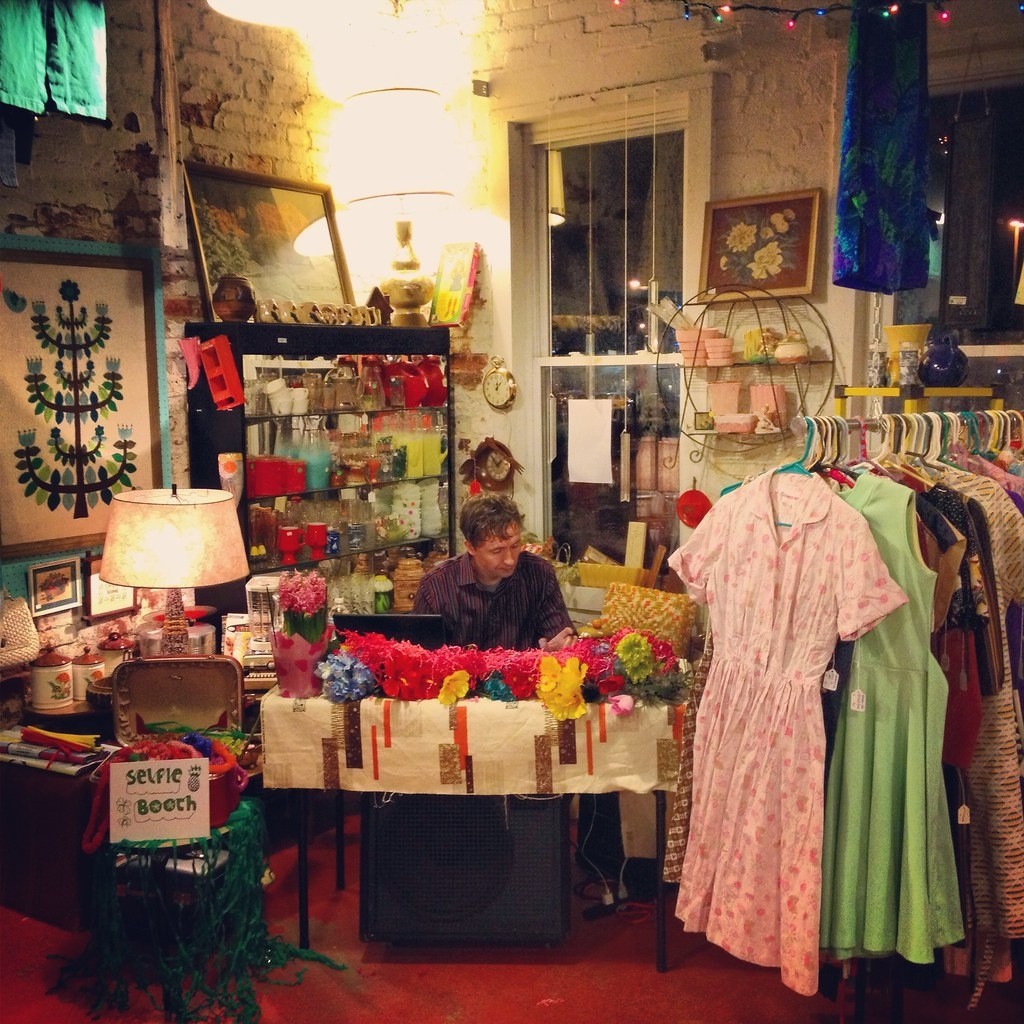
[271,566,683,722]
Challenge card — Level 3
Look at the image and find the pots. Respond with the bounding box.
[139,620,217,656]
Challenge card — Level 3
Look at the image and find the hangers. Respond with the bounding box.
[720,408,1024,529]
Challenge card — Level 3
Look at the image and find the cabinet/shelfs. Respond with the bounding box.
[183,322,456,652]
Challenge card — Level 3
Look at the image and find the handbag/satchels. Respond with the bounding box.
[1,589,40,672]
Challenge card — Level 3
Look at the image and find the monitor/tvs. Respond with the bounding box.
[334,614,445,653]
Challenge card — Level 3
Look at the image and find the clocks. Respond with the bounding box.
[482,353,516,412]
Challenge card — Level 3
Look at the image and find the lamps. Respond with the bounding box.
[98,483,250,658]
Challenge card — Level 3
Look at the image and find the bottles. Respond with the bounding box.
[338,355,359,379]
[362,356,385,410]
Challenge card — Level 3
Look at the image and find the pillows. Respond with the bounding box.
[579,583,698,659]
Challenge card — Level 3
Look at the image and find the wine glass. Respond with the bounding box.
[277,527,308,565]
[306,522,328,560]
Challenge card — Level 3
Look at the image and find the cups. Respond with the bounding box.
[96,632,135,677]
[244,373,448,486]
[254,299,378,326]
[72,646,105,700]
[28,644,73,709]
[250,499,376,568]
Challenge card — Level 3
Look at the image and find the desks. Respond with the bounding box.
[261,666,699,973]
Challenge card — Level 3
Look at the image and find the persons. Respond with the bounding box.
[754,405,774,433]
[411,491,651,889]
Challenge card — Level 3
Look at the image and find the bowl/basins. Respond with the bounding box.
[393,478,442,539]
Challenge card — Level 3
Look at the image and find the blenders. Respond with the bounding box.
[244,577,280,689]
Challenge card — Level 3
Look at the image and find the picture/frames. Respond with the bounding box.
[28,557,83,616]
[181,161,357,323]
[81,549,141,627]
[697,185,824,305]
